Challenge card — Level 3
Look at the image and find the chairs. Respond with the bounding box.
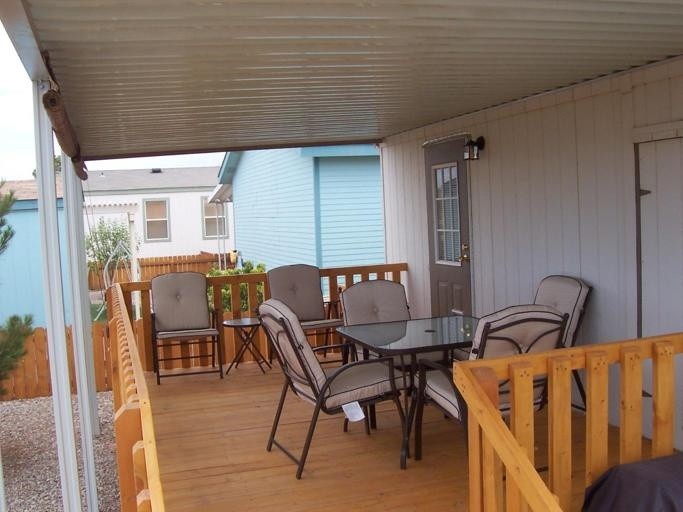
[147,269,225,387]
[265,264,345,370]
[255,274,593,479]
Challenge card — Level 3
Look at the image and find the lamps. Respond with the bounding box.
[462,135,487,161]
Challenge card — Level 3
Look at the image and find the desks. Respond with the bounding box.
[224,317,272,374]
